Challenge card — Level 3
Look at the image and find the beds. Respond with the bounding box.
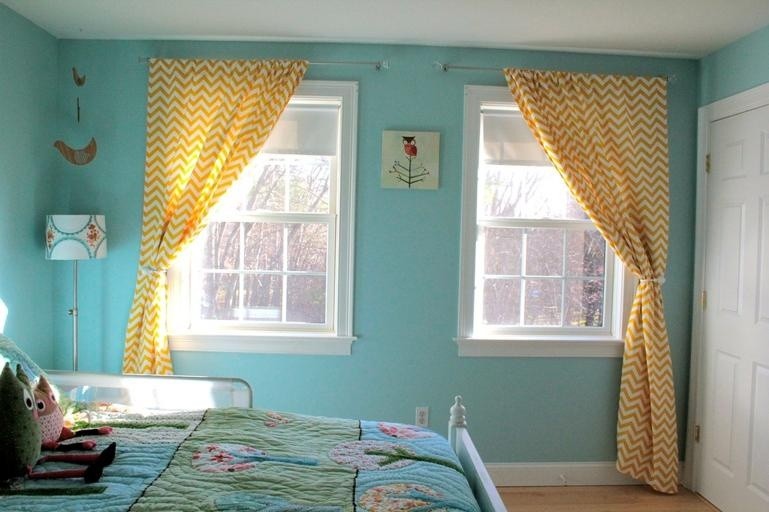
[0,397,508,512]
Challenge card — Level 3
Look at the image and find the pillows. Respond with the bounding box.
[1,361,116,483]
[32,374,113,452]
[0,331,64,406]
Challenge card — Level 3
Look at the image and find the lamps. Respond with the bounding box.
[45,214,107,371]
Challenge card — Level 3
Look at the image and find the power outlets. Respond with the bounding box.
[415,406,428,428]
